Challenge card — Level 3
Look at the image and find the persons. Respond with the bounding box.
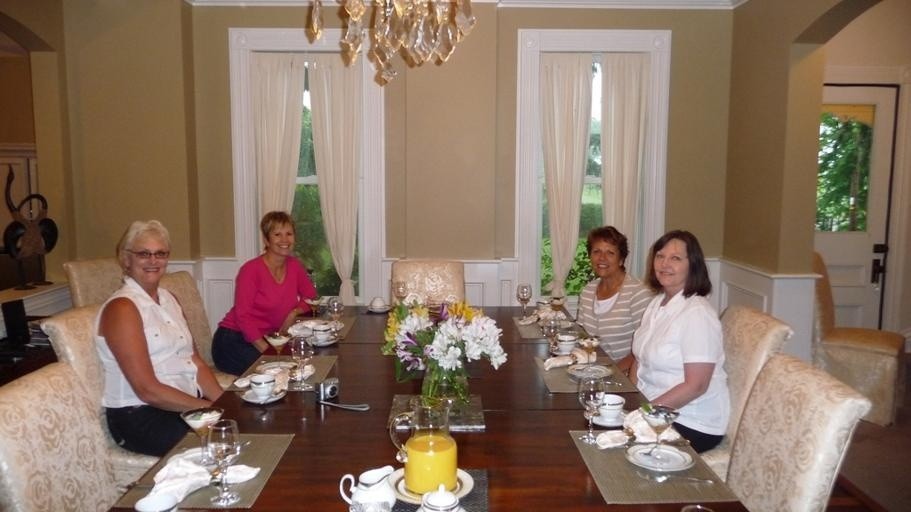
[575,226,652,372]
[211,212,318,375]
[628,229,729,453]
[93,219,224,457]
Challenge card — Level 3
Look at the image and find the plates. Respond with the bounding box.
[589,409,627,428]
[621,443,697,473]
[256,362,298,373]
[236,388,288,406]
[385,467,477,505]
[366,307,392,314]
[288,318,342,347]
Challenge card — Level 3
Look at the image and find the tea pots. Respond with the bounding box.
[339,463,399,511]
[387,396,459,495]
[416,484,470,512]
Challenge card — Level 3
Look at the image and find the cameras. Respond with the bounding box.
[319,378,340,401]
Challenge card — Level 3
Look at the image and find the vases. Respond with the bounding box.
[420,364,472,405]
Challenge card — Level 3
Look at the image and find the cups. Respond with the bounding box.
[252,375,277,399]
[597,392,625,422]
[394,280,408,301]
[682,504,714,511]
[370,297,386,310]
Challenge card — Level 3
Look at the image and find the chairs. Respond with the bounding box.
[1,362,155,511]
[811,251,903,426]
[63,256,125,310]
[700,302,795,482]
[728,354,871,512]
[389,258,466,305]
[41,301,165,469]
[154,271,238,390]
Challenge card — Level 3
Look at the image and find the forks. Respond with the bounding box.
[633,468,718,490]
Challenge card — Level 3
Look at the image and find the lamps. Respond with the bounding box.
[304,0,477,87]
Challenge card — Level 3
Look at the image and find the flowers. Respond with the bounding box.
[380,300,507,370]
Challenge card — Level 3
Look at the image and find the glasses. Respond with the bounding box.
[127,249,169,259]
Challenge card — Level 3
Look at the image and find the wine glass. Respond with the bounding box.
[263,331,291,369]
[575,377,611,448]
[635,402,678,460]
[515,282,613,379]
[180,407,242,506]
[302,294,343,323]
[290,339,314,393]
